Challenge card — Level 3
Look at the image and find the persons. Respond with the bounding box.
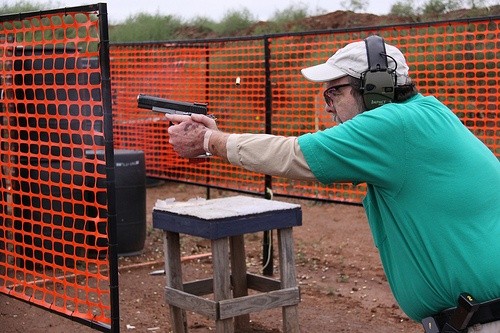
[165,40,500,333]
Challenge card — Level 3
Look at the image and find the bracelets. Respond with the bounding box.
[202,129,213,156]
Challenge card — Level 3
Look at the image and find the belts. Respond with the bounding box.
[431,298,500,331]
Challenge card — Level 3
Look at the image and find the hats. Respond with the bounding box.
[299,41,410,84]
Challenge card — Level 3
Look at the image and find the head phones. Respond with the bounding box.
[357,35,396,111]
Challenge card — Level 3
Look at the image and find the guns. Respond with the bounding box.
[136,94,214,159]
